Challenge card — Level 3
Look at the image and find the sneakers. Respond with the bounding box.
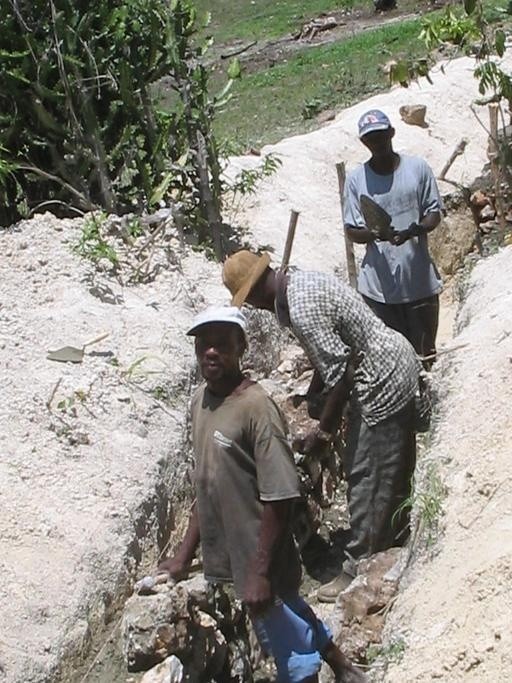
[318,571,354,603]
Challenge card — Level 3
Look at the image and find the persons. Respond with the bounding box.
[155,305,368,683]
[341,108,446,435]
[220,248,424,603]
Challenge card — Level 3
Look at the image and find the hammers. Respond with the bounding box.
[134,558,200,595]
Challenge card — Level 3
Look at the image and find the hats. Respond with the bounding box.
[185,307,247,337]
[223,250,271,307]
[358,109,392,138]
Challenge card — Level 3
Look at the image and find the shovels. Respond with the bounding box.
[360,195,396,245]
[47,333,108,362]
